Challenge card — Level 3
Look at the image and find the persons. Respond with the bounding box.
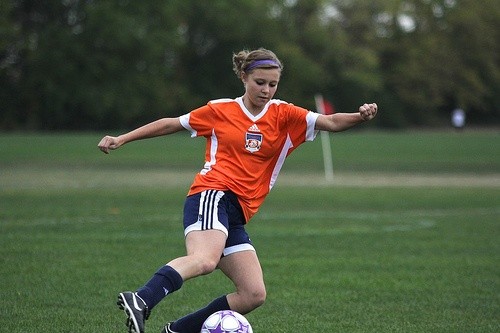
[96,49,378,333]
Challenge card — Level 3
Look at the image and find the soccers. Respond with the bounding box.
[200,310,253,333]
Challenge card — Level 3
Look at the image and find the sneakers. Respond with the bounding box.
[160,321,179,333]
[116,291,150,333]
